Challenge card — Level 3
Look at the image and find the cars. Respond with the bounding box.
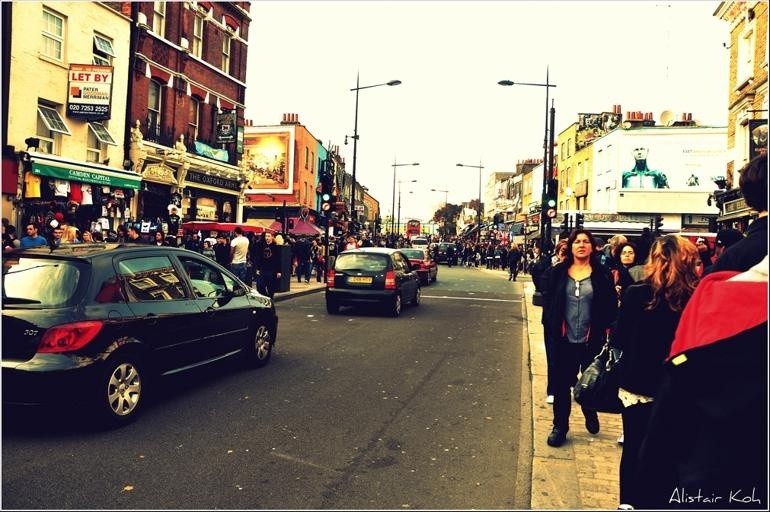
[326,248,422,319]
[5,236,280,429]
[396,248,439,286]
[430,242,458,264]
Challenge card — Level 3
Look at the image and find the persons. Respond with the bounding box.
[713,151,768,271]
[2,229,767,508]
[623,145,670,189]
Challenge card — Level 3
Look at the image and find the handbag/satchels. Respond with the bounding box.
[573,333,623,415]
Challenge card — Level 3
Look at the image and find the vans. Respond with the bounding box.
[410,236,429,249]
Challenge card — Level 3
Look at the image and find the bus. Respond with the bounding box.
[406,220,420,240]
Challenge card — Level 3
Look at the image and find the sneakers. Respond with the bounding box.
[548,425,567,446]
[546,394,554,404]
[581,404,599,434]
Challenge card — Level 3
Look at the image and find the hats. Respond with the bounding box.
[715,229,745,247]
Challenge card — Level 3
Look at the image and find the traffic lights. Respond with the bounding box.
[288,219,294,229]
[576,213,584,231]
[560,214,567,239]
[319,173,332,206]
[546,179,558,207]
[275,206,284,223]
[655,215,664,234]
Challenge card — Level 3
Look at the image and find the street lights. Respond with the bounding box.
[456,160,486,242]
[391,160,422,237]
[430,188,448,238]
[344,73,401,233]
[497,77,555,263]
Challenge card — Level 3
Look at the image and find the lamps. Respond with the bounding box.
[25,137,41,151]
[123,159,134,171]
